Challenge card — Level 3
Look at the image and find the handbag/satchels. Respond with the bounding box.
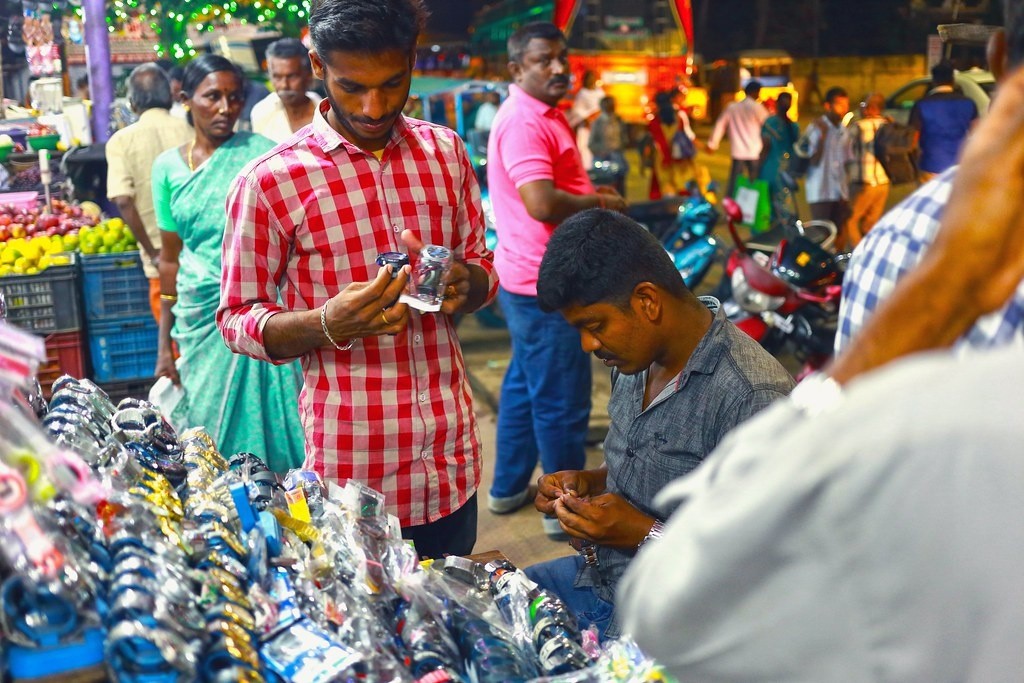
[736,187,757,223]
[639,135,654,166]
[672,131,694,159]
[594,153,627,178]
[787,118,828,176]
[731,173,771,231]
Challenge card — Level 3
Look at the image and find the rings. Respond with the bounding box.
[381,312,391,326]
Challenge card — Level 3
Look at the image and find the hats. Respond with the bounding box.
[933,64,952,81]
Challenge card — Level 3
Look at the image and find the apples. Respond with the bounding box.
[0,198,99,244]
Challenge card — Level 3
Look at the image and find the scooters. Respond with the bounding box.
[654,130,857,381]
[585,159,683,242]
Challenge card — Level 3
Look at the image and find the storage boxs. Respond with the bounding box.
[84,322,160,386]
[0,191,38,210]
[31,76,64,115]
[0,250,83,334]
[78,250,156,330]
[36,331,84,403]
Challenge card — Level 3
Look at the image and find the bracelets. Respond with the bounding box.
[599,193,607,210]
[321,298,356,350]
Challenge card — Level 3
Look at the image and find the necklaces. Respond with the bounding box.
[188,141,198,173]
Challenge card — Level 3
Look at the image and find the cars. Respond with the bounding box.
[851,72,995,126]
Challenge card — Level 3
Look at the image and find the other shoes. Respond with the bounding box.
[488,481,537,512]
[543,513,567,535]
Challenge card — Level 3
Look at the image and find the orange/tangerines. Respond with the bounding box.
[0,218,139,280]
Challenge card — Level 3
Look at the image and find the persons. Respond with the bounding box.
[523,207,797,649]
[834,0,1024,368]
[78,37,326,475]
[215,1,498,556]
[612,62,1024,683]
[488,21,627,532]
[456,61,980,248]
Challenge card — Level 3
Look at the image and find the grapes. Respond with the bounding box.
[10,161,68,193]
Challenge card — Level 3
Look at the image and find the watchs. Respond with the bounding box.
[0,298,673,683]
[635,519,664,551]
[419,243,453,304]
[374,251,411,312]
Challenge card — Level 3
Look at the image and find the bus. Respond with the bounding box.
[697,48,798,127]
[468,0,705,145]
